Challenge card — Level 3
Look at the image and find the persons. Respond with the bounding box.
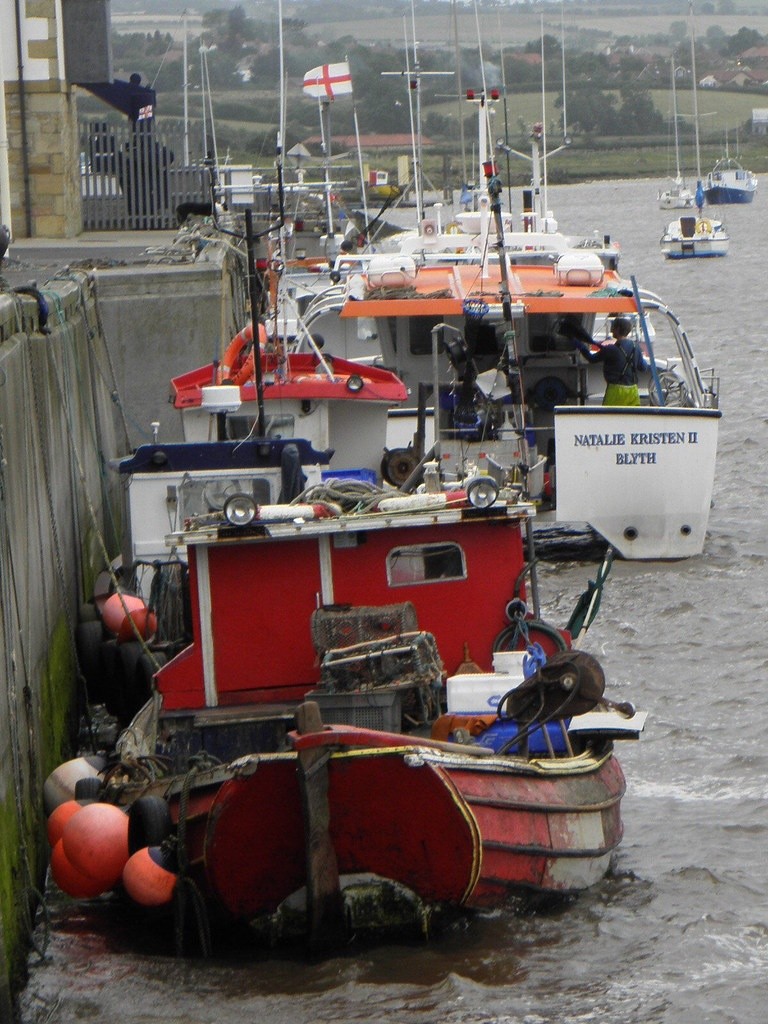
[572,319,648,406]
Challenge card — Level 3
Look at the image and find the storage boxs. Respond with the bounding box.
[493,650,537,677]
[446,672,525,713]
[306,690,402,735]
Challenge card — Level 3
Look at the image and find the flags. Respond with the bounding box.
[303,62,353,98]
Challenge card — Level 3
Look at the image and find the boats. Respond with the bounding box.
[103,459,648,962]
[659,217,729,258]
[264,204,620,342]
[271,262,723,562]
[169,350,410,496]
[101,438,381,717]
[703,157,758,206]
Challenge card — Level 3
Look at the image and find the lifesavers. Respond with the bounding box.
[216,320,268,385]
[190,501,340,525]
[379,491,506,510]
[696,218,712,235]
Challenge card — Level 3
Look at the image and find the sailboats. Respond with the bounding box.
[656,56,696,209]
